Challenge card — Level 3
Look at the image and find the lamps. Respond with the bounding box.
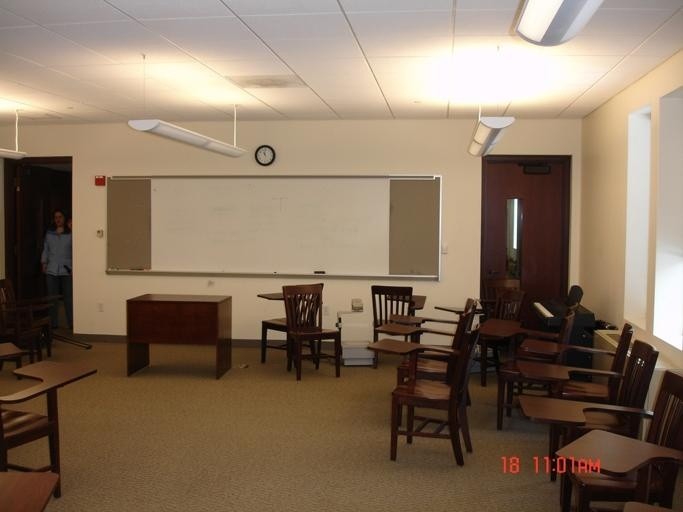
[511,0,605,49]
[124,50,247,159]
[0,108,28,162]
[463,98,518,160]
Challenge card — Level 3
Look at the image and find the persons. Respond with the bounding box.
[66,217,72,233]
[40,208,72,334]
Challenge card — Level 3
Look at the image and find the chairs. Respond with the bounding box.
[0,275,100,512]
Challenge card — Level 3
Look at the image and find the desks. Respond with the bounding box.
[124,291,233,382]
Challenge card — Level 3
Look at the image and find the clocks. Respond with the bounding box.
[254,144,276,167]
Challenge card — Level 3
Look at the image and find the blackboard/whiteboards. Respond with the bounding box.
[314,271,325,274]
[106,174,442,281]
